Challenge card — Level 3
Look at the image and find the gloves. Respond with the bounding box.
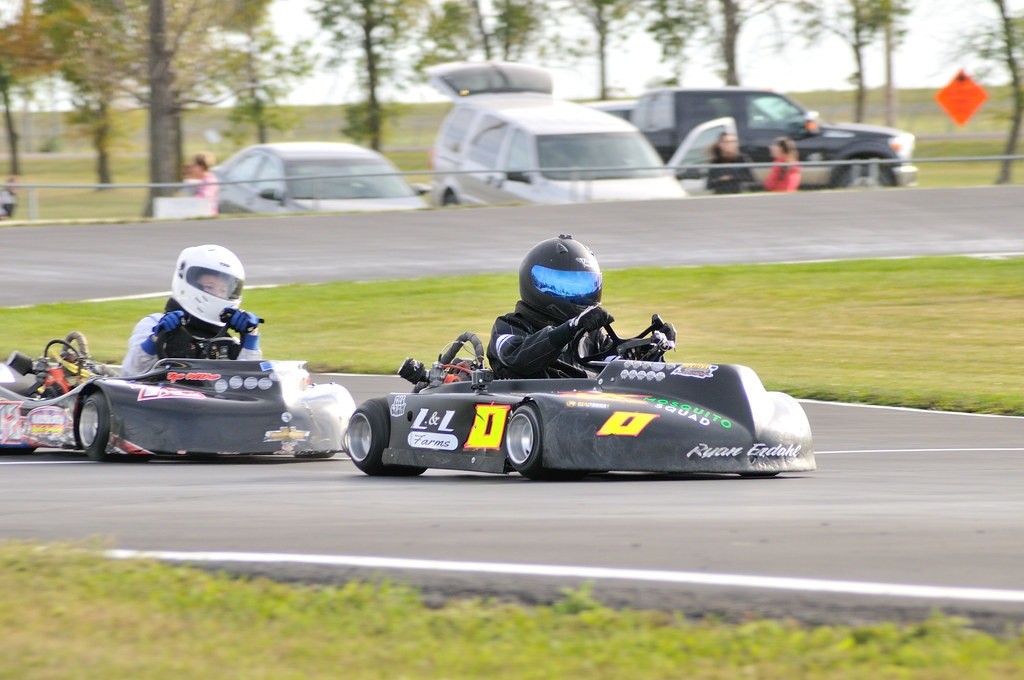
[568,305,615,333]
[650,323,677,350]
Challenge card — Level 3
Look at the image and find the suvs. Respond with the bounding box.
[631,89,916,189]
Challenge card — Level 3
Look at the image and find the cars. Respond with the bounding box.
[577,100,636,124]
[184,143,436,215]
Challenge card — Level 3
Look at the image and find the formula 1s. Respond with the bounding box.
[344,310,818,483]
[1,331,358,464]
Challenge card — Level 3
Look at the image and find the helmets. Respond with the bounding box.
[170,244,245,326]
[518,234,602,321]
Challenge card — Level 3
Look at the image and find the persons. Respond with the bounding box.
[705,131,755,194]
[487,233,677,379]
[761,135,801,190]
[0,150,220,221]
[118,244,265,379]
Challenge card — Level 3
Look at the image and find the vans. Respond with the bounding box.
[420,57,688,209]
